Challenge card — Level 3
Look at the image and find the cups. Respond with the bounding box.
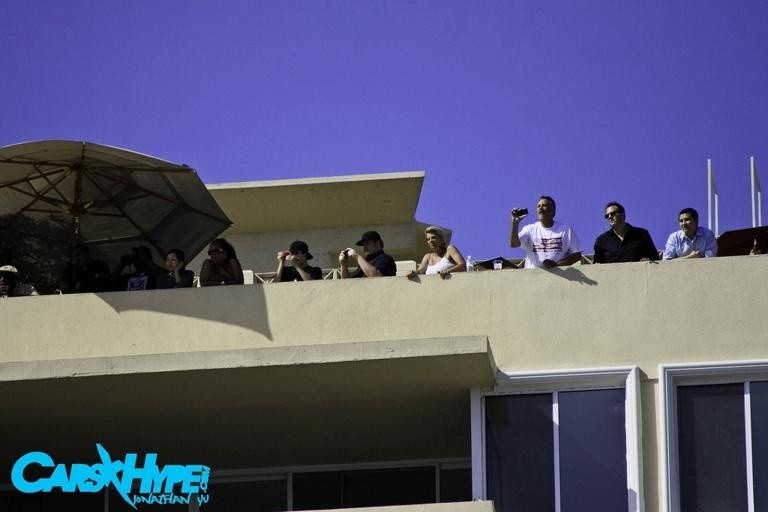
[493,260,504,270]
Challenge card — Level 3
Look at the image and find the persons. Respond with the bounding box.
[158,248,195,290]
[594,201,660,264]
[661,208,718,259]
[337,228,398,278]
[509,194,583,267]
[198,238,244,284]
[407,225,467,281]
[273,240,322,281]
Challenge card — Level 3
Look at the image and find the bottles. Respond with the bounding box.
[749,237,763,255]
[466,256,473,272]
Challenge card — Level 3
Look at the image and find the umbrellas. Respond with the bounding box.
[0,139,235,284]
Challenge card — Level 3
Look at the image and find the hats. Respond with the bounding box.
[289,240,313,260]
[355,231,381,246]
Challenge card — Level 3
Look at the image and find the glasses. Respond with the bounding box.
[604,210,619,219]
[208,248,222,256]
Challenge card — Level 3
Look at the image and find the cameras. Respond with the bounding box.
[512,208,527,216]
